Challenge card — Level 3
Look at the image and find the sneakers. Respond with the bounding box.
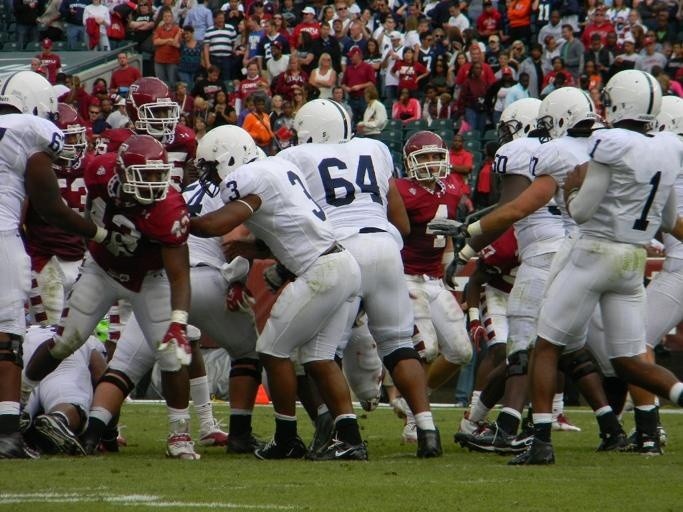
[0,405,128,458]
[454,405,582,465]
[198,429,228,446]
[393,396,443,458]
[226,410,369,461]
[166,432,202,463]
[595,423,669,456]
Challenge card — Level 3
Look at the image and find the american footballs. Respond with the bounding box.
[564,163,590,200]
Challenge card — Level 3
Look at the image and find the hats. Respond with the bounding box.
[643,37,654,46]
[270,41,282,49]
[488,35,499,44]
[346,46,360,58]
[391,31,402,40]
[302,7,315,16]
[42,39,52,48]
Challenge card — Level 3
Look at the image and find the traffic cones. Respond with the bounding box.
[250,385,269,405]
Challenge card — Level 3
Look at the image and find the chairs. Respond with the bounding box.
[269,108,497,200]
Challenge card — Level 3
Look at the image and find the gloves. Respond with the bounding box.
[155,310,193,366]
[262,263,293,294]
[442,243,475,289]
[427,218,483,241]
[92,226,137,260]
[467,307,489,352]
[225,280,256,315]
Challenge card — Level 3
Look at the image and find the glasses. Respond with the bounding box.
[338,7,347,10]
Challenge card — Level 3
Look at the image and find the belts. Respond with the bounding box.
[319,244,346,256]
[360,227,388,233]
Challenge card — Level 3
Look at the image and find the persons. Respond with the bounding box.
[1,1,683,467]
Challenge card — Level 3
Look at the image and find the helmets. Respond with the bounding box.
[404,130,451,182]
[496,69,683,147]
[194,124,260,198]
[0,71,180,208]
[292,98,354,144]
